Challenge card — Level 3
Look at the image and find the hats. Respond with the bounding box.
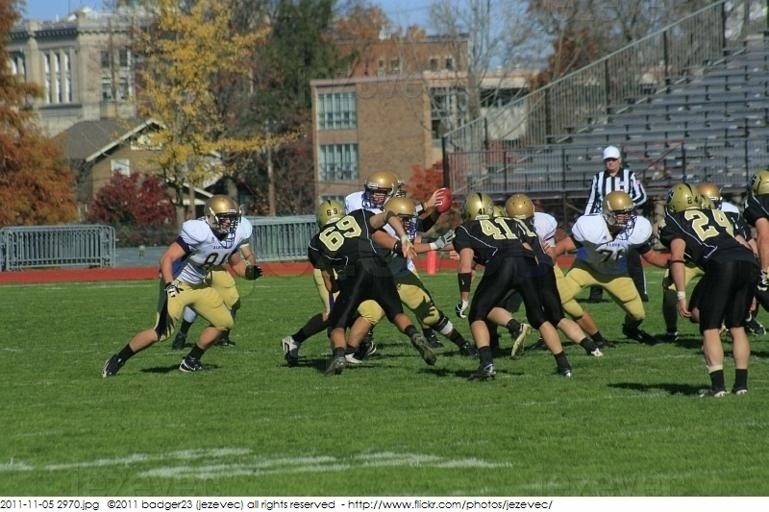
[603,145,620,160]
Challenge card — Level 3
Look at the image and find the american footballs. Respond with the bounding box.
[435,185,453,213]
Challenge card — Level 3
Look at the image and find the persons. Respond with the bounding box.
[281,187,456,365]
[652,217,766,343]
[657,183,761,399]
[344,174,476,359]
[102,196,263,380]
[486,193,615,356]
[485,207,604,359]
[584,145,649,304]
[696,183,758,337]
[452,193,573,382]
[743,170,769,313]
[354,180,445,357]
[309,200,437,376]
[524,191,672,354]
[171,200,256,351]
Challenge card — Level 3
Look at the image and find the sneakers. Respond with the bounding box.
[467,361,496,382]
[747,315,766,336]
[595,338,616,350]
[412,332,436,366]
[655,331,679,342]
[558,368,572,378]
[510,323,532,360]
[731,386,748,394]
[102,353,125,378]
[695,386,726,400]
[527,337,551,354]
[172,334,191,350]
[214,336,236,348]
[281,336,301,364]
[324,357,344,376]
[426,333,444,349]
[178,356,204,373]
[622,323,648,343]
[588,347,603,358]
[345,354,367,367]
[460,341,477,358]
[367,342,376,356]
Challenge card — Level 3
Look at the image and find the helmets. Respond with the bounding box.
[696,182,720,201]
[362,170,398,209]
[746,169,769,202]
[493,205,506,217]
[504,193,535,220]
[231,199,241,222]
[464,191,493,221]
[203,194,238,235]
[384,196,416,233]
[602,191,637,232]
[700,193,715,210]
[663,182,703,216]
[386,169,406,198]
[316,200,346,230]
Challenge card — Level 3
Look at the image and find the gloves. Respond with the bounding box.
[164,282,184,297]
[757,270,769,297]
[456,300,468,319]
[433,229,456,249]
[245,264,263,281]
